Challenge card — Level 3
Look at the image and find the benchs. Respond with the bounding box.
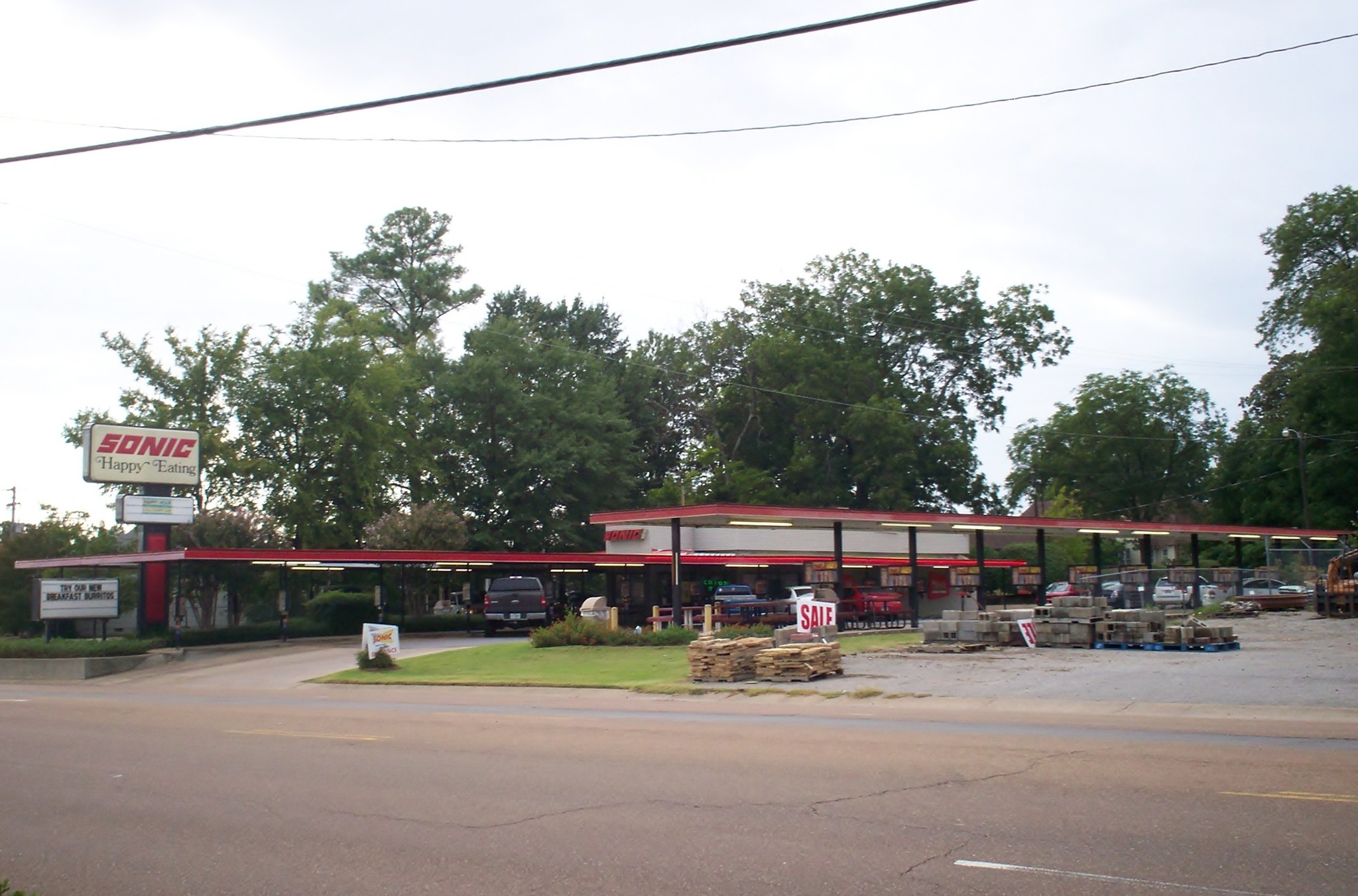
[646,611,917,631]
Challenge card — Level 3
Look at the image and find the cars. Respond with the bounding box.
[1100,580,1154,608]
[831,583,901,626]
[1200,578,1289,608]
[767,585,815,615]
[1035,580,1097,607]
[1153,574,1213,609]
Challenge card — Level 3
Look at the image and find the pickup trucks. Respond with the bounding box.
[709,584,769,618]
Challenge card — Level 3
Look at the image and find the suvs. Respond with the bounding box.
[484,575,548,638]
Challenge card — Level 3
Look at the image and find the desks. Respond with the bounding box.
[660,596,900,630]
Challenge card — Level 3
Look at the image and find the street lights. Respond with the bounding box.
[1279,427,1317,558]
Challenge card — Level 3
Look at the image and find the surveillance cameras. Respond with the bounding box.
[1282,431,1289,437]
